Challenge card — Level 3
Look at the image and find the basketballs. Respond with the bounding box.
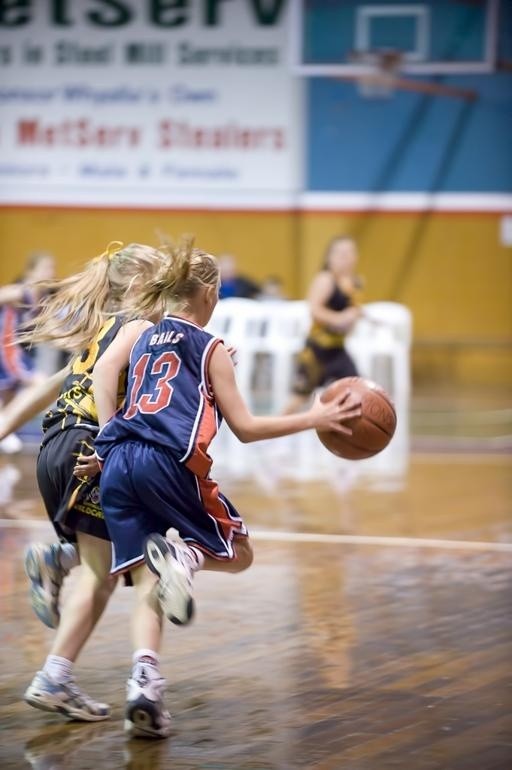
[314,377,396,460]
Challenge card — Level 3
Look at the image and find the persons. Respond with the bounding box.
[0,253,63,456]
[93,244,363,738]
[0,244,240,723]
[279,236,366,417]
[216,253,265,298]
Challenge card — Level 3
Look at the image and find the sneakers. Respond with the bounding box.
[22,669,112,721]
[142,530,197,627]
[0,433,23,453]
[25,541,69,630]
[124,662,173,740]
